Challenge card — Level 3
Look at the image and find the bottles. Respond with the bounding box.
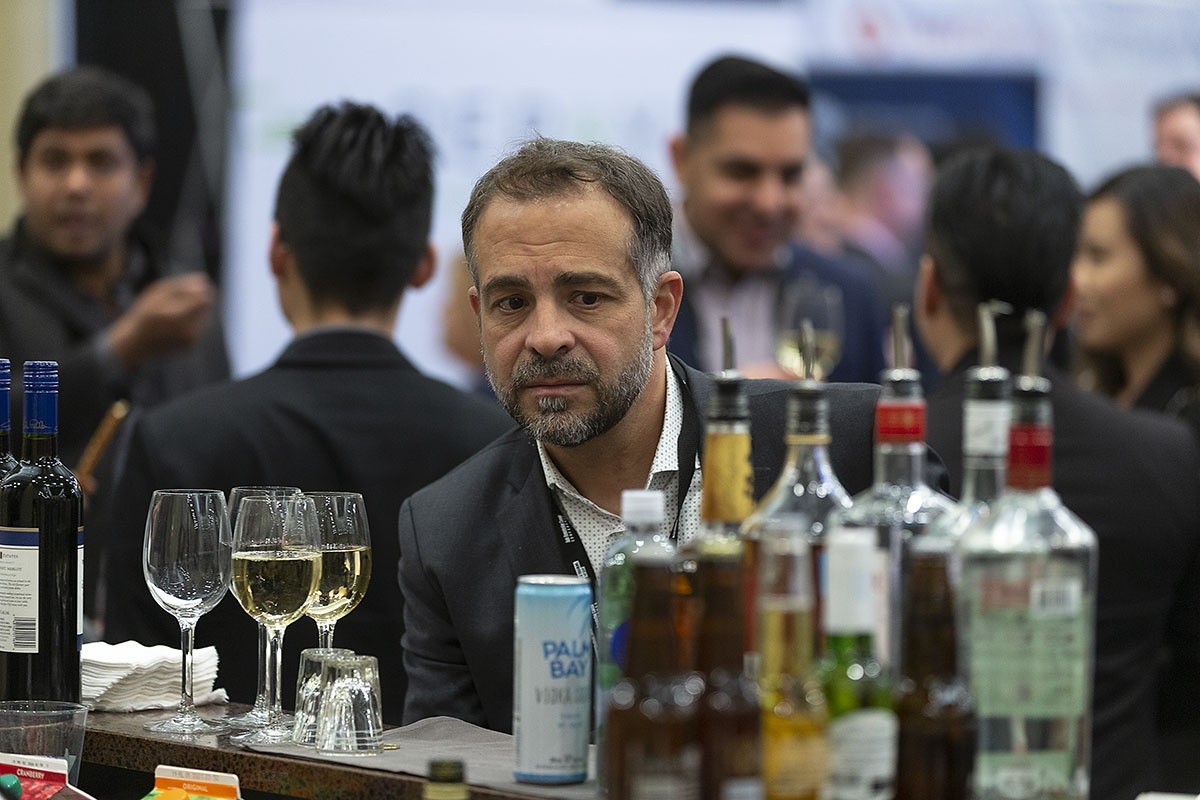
[422,759,469,800]
[592,302,1098,799]
[0,358,84,714]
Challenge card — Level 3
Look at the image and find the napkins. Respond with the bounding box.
[80,641,227,709]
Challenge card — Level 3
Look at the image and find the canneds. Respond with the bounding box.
[512,575,592,785]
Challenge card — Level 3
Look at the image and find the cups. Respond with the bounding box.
[293,648,385,756]
[0,700,89,788]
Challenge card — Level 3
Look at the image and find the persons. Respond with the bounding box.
[395,140,952,743]
[104,105,524,729]
[1,66,232,636]
[445,53,1200,799]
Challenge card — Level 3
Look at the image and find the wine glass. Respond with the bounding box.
[140,487,372,747]
